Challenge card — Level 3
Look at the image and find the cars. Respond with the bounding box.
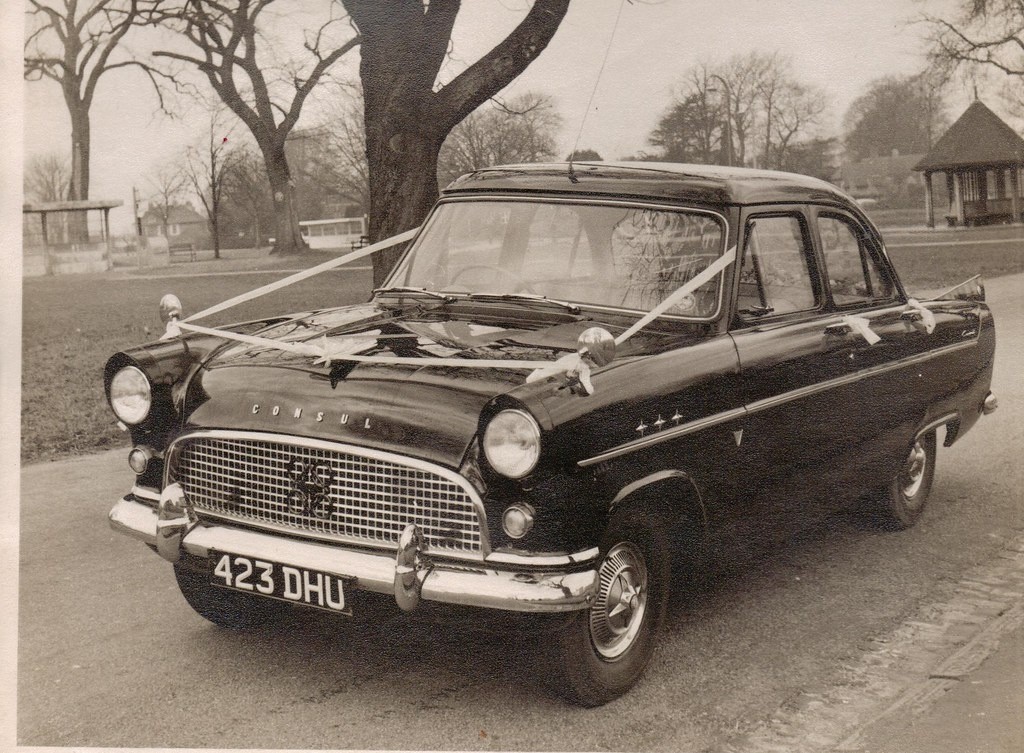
[101,161,999,707]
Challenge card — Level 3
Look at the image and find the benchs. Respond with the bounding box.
[348,235,370,252]
[168,243,197,263]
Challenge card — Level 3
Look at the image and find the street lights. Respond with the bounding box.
[704,74,732,165]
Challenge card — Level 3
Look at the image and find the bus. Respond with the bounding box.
[295,217,370,251]
